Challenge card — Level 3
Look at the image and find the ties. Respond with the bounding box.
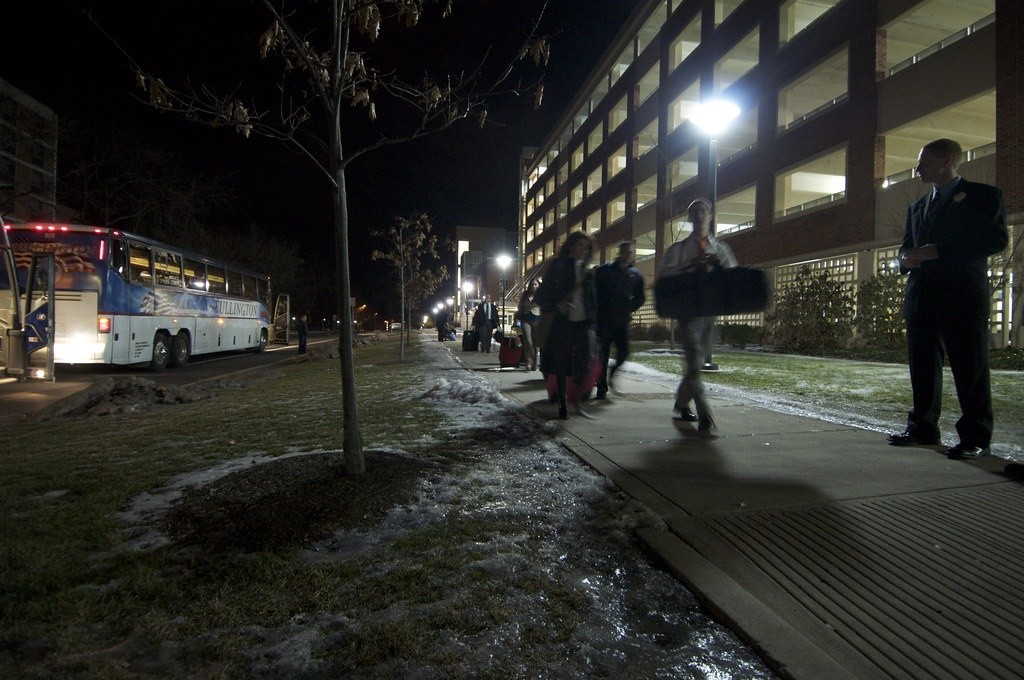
[929,190,941,215]
[485,303,488,321]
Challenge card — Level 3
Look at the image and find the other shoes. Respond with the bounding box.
[597,390,607,400]
[559,399,568,411]
[698,414,715,433]
[674,400,697,421]
[527,363,532,371]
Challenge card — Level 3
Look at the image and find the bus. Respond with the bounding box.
[0,217,272,384]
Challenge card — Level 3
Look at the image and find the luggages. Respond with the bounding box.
[463,326,479,351]
[499,325,522,367]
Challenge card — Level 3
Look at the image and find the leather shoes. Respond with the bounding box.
[888,430,940,445]
[947,443,990,459]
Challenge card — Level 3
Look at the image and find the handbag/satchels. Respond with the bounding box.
[655,264,772,319]
[530,318,554,348]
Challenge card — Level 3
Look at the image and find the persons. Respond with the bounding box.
[437,305,448,341]
[298,315,307,355]
[510,232,645,421]
[657,199,735,431]
[887,139,1008,460]
[472,294,500,353]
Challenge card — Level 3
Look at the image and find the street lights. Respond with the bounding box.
[462,283,474,331]
[687,97,739,371]
[497,253,513,334]
[420,303,444,329]
[447,298,454,329]
